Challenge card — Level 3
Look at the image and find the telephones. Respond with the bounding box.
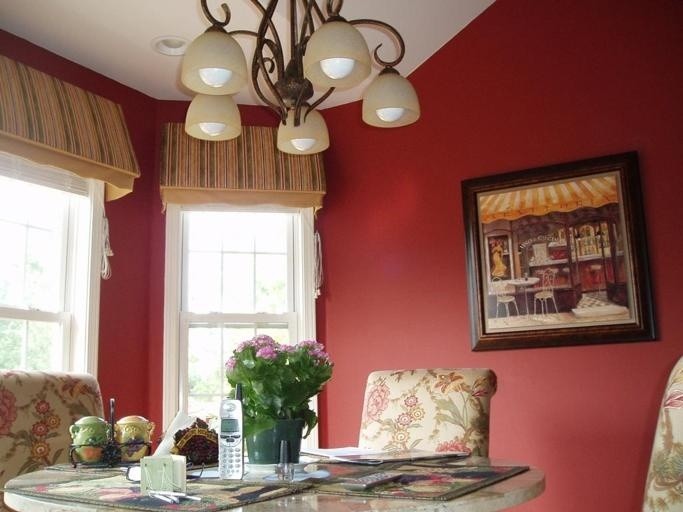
[218,383,244,481]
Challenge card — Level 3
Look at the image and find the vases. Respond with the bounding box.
[245,416,305,465]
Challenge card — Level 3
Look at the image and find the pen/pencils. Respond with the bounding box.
[149,490,201,503]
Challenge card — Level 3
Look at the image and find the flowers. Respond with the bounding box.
[227,335,335,439]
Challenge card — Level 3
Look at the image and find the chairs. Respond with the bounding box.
[642,357,682,512]
[360,368,498,466]
[0,369,104,512]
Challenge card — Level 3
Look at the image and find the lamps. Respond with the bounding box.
[181,0,420,157]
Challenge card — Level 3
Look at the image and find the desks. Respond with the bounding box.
[4,454,548,512]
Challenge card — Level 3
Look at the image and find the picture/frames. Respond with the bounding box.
[459,150,660,353]
